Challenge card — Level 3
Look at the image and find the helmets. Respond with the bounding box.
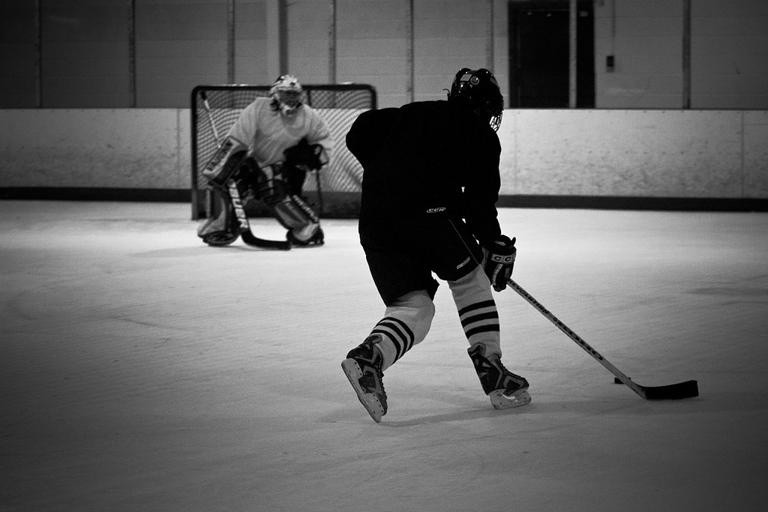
[268,74,306,119]
[447,67,504,132]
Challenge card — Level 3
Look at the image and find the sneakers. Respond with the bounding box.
[346,334,388,417]
[467,342,529,396]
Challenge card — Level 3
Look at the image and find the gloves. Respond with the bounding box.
[480,234,516,293]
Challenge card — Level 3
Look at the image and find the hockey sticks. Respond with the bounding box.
[198,87,291,252]
[505,278,699,401]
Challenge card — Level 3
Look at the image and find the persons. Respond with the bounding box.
[192,72,334,248]
[343,63,529,419]
